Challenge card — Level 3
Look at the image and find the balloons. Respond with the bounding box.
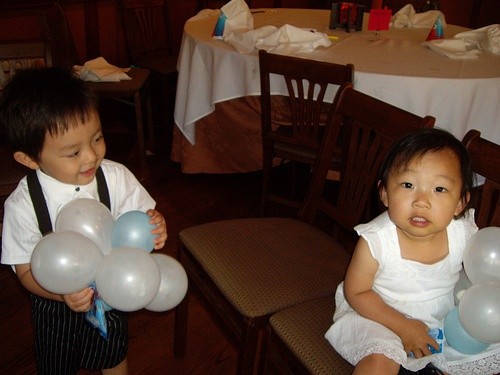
[29,198,189,313]
[443,227,500,355]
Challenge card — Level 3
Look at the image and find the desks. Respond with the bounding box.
[184,7,500,172]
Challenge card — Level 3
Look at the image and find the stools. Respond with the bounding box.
[86,68,155,150]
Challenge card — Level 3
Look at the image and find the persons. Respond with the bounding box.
[0,65,168,375]
[324,128,500,375]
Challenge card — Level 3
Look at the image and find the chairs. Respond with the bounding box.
[118,0,178,120]
[34,3,80,64]
[173,82,436,375]
[258,50,355,219]
[259,130,500,375]
